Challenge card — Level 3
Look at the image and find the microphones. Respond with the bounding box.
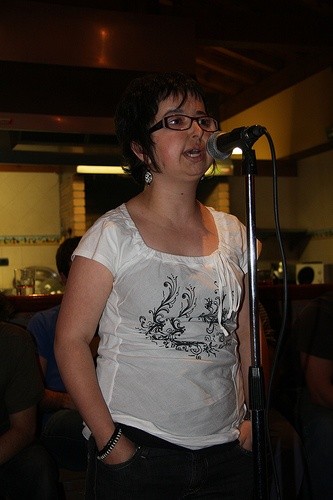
[206,125,266,160]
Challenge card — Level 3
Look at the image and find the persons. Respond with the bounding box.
[0,236,99,500]
[298,267,314,285]
[277,294,333,500]
[55,72,263,500]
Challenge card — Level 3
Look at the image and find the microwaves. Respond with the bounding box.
[295,262,333,284]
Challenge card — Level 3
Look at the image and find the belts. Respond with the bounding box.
[118,424,240,452]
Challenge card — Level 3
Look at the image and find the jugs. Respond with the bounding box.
[15,267,35,295]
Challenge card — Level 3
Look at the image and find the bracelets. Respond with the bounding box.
[96,426,122,461]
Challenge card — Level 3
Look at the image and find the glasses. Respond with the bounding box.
[148,115,221,134]
